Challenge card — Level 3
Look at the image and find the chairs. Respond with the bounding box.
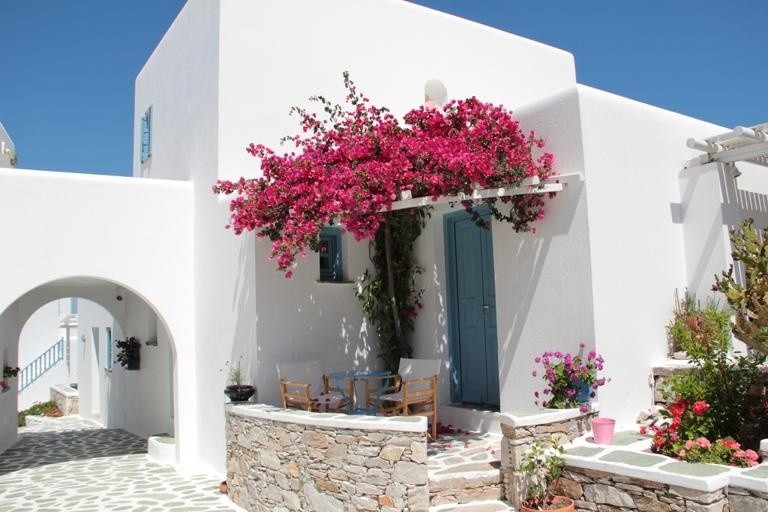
[365,358,442,442]
[279,379,350,412]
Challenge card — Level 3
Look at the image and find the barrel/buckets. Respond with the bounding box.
[590,418,616,446]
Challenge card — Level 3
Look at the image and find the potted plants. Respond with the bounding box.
[219,355,256,402]
[517,434,574,512]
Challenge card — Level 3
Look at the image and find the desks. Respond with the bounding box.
[322,371,391,414]
